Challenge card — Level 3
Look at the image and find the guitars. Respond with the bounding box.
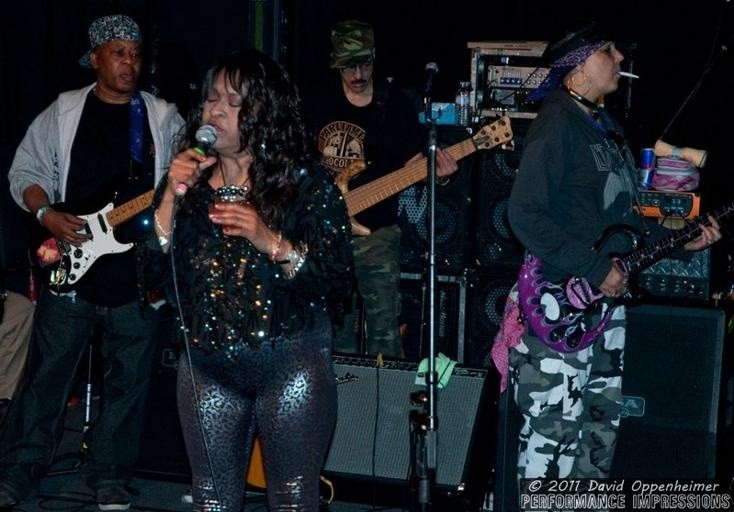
[334,115,515,239]
[520,196,733,354]
[24,190,156,294]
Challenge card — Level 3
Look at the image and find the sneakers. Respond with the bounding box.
[95,485,134,511]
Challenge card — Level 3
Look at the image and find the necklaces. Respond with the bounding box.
[216,158,251,201]
[568,90,597,110]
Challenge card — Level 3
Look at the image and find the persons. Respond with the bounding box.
[143,48,351,510]
[1,13,188,510]
[308,22,457,358]
[508,20,722,508]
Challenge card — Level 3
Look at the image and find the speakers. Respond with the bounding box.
[322,354,489,502]
[389,123,530,371]
[610,305,726,495]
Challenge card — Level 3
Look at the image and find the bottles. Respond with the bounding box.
[454,77,472,127]
[499,71,524,87]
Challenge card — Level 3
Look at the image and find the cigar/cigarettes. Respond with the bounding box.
[618,71,639,80]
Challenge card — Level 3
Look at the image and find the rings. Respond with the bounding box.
[612,291,618,298]
[708,240,715,245]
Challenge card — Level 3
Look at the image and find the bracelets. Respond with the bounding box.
[281,248,303,278]
[36,206,50,224]
[270,232,282,263]
[153,209,176,237]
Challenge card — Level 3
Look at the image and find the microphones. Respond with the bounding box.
[172,124,218,198]
[421,61,440,92]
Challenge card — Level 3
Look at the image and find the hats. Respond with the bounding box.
[77,13,144,71]
[523,21,616,102]
[327,18,377,69]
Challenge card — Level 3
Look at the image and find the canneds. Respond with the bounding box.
[638,148,657,190]
